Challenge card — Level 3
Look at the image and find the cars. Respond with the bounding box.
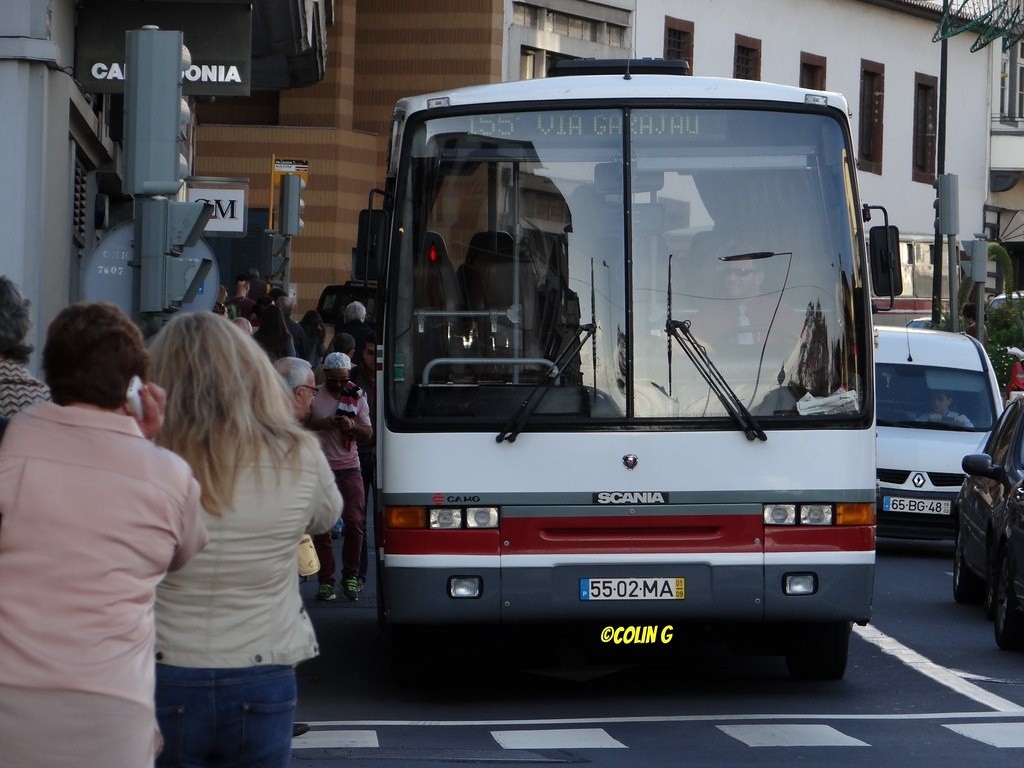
[985,290,1024,321]
[906,315,965,331]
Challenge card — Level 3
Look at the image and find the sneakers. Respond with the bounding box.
[314,584,336,600]
[338,574,359,601]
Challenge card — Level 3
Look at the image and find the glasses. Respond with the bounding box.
[723,267,762,278]
[326,376,351,383]
[294,384,319,396]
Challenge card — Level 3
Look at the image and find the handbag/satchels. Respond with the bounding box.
[296,531,321,580]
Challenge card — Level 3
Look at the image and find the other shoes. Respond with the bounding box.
[292,723,310,737]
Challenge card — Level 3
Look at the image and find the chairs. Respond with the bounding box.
[890,372,925,402]
[924,390,962,411]
[413,228,762,418]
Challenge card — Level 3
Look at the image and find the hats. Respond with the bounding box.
[322,352,352,370]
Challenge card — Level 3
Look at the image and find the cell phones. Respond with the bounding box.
[126,375,145,422]
[334,417,344,421]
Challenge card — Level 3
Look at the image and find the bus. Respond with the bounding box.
[355,71,904,685]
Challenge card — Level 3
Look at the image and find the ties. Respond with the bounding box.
[737,305,754,345]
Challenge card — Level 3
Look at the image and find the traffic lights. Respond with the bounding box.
[427,235,443,315]
[120,26,193,199]
[961,239,987,283]
[278,175,309,238]
[134,198,214,315]
[265,232,291,278]
[933,174,960,237]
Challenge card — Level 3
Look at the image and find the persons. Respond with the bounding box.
[988,295,995,303]
[915,390,974,428]
[147,311,345,768]
[0,273,54,448]
[0,302,211,768]
[689,249,809,347]
[212,276,377,602]
[963,304,988,349]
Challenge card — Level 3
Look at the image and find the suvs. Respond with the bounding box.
[953,393,1023,651]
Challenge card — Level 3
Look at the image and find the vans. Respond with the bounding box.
[870,325,1023,548]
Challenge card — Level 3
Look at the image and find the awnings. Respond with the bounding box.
[998,209,1024,242]
